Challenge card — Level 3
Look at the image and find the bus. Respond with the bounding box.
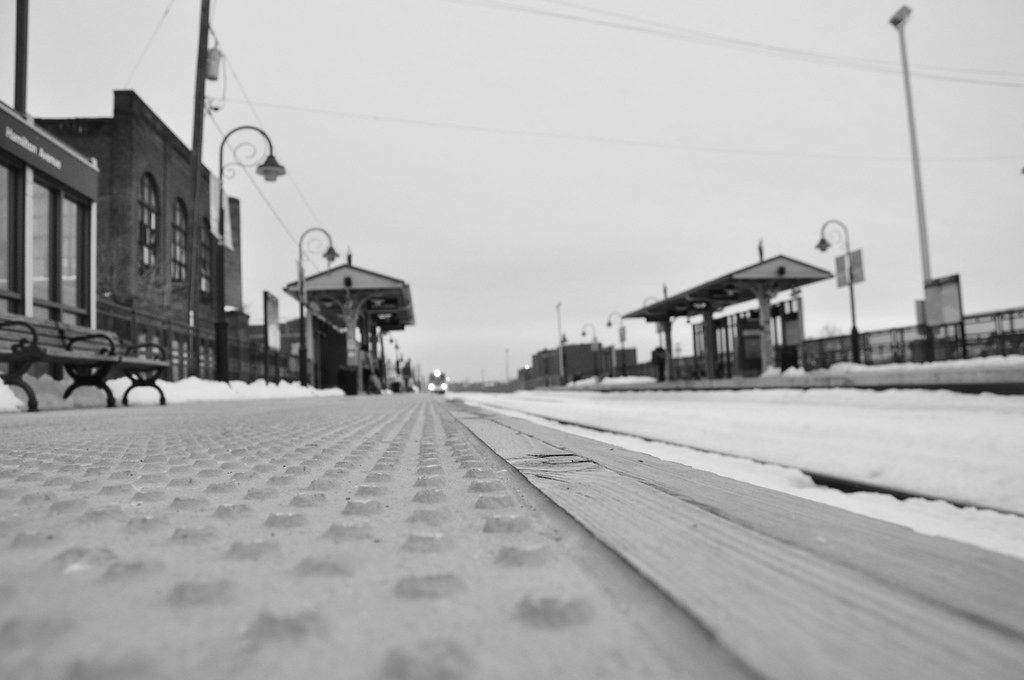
[429,372,446,394]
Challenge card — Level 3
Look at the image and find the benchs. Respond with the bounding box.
[0,311,171,412]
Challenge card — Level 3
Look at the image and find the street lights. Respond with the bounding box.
[581,323,598,375]
[889,5,935,361]
[816,220,860,363]
[298,227,339,386]
[607,312,627,376]
[216,125,287,381]
